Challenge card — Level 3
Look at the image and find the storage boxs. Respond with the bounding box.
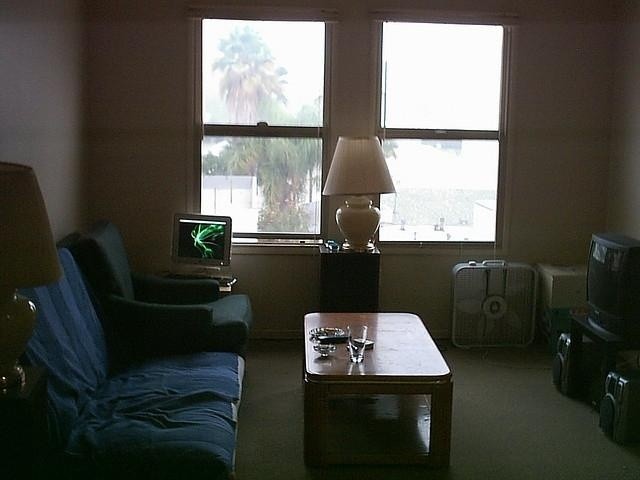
[526,261,590,308]
[541,303,582,353]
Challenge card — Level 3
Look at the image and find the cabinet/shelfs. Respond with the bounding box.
[316,240,381,312]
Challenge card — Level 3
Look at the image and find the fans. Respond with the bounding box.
[449,258,543,354]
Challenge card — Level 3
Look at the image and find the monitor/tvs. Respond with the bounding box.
[585,233,639,336]
[172,213,231,271]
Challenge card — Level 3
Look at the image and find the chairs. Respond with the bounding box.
[0,246,248,477]
[61,218,254,364]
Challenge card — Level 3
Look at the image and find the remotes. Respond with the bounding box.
[319,335,348,343]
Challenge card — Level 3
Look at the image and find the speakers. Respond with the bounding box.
[552,332,577,394]
[598,369,640,432]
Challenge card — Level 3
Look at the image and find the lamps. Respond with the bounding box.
[322,135,397,250]
[0,158,63,396]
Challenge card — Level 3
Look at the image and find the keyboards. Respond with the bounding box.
[167,274,239,287]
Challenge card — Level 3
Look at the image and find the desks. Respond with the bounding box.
[566,309,640,411]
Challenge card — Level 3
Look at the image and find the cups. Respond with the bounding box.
[347,325,369,363]
